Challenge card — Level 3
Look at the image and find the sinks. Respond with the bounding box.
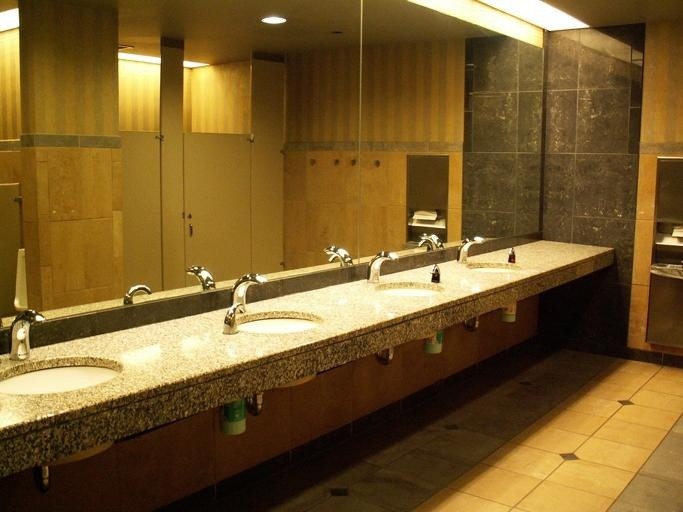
[372,280,447,298]
[0,354,124,398]
[231,309,325,335]
[465,260,524,274]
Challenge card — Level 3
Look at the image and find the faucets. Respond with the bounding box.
[456,234,485,262]
[8,309,45,360]
[185,266,217,290]
[124,282,153,305]
[366,249,400,284]
[322,245,353,266]
[229,271,268,313]
[417,232,444,252]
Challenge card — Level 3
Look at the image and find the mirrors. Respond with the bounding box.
[0,0,547,328]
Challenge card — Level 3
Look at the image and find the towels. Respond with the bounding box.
[408,208,446,230]
[655,226,683,246]
